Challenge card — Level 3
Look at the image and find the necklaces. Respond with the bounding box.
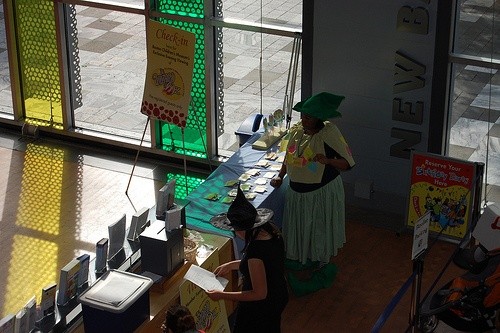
[298,130,316,158]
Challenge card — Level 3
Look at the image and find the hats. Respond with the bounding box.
[292,91,346,121]
[208,181,274,231]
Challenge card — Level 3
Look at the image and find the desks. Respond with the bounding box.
[72,229,239,333]
[183,131,288,284]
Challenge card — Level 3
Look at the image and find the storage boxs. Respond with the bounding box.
[57,257,80,306]
[42,283,57,316]
[77,268,154,333]
[96,238,108,270]
[78,254,90,285]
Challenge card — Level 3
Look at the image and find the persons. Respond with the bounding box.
[166,304,206,333]
[205,186,288,333]
[269,91,355,264]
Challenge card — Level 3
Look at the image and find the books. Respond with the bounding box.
[0,181,177,333]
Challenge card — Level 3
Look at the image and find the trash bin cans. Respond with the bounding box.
[235,113,272,147]
